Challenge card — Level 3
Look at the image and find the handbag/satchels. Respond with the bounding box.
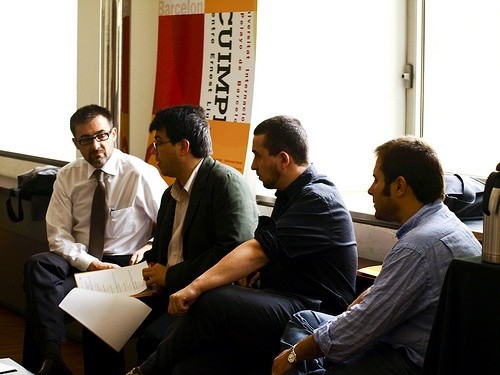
[444,174,488,221]
[5,165,59,223]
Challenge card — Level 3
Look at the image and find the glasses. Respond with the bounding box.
[75,128,113,146]
[153,137,192,153]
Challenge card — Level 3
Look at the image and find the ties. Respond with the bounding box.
[87,169,105,261]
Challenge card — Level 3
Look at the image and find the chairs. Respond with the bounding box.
[420,258,500,375]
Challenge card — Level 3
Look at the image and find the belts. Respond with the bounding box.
[103,257,129,263]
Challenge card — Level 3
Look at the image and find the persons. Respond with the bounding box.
[272,135,483,375]
[22,105,170,375]
[126,115,358,374]
[81,105,259,375]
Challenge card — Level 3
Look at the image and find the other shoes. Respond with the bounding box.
[125,366,142,375]
[35,359,73,375]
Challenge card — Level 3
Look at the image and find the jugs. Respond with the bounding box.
[482,162,500,265]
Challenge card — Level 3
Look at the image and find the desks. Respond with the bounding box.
[356,263,383,296]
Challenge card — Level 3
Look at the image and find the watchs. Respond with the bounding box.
[287,344,298,365]
